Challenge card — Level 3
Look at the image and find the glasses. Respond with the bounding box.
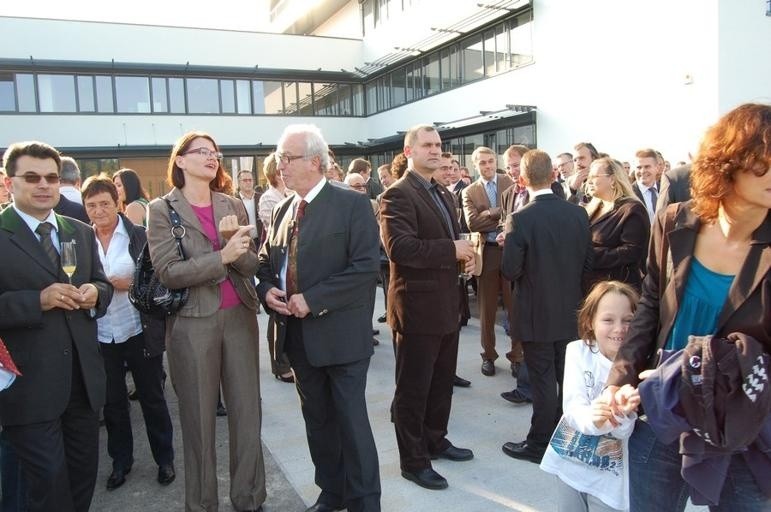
[14,172,60,183]
[181,147,223,160]
[273,151,307,163]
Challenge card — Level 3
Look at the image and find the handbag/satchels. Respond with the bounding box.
[128,243,188,314]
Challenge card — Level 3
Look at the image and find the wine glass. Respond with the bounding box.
[59,241,79,287]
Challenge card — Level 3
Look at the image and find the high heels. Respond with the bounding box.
[129,370,166,401]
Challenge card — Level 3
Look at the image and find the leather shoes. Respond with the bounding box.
[107,466,129,491]
[158,460,174,485]
[432,446,473,462]
[275,371,295,383]
[511,362,521,378]
[502,440,547,464]
[402,465,448,490]
[452,377,471,387]
[483,359,497,377]
[503,388,532,402]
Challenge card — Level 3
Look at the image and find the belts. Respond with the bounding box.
[485,242,499,247]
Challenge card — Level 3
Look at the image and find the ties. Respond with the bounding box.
[286,199,307,305]
[487,182,498,239]
[36,222,61,268]
[649,188,658,211]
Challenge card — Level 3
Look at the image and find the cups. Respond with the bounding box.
[458,229,481,276]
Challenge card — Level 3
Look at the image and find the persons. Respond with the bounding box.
[2,126,407,512]
[597,103,770,510]
[433,141,701,509]
[377,124,473,490]
